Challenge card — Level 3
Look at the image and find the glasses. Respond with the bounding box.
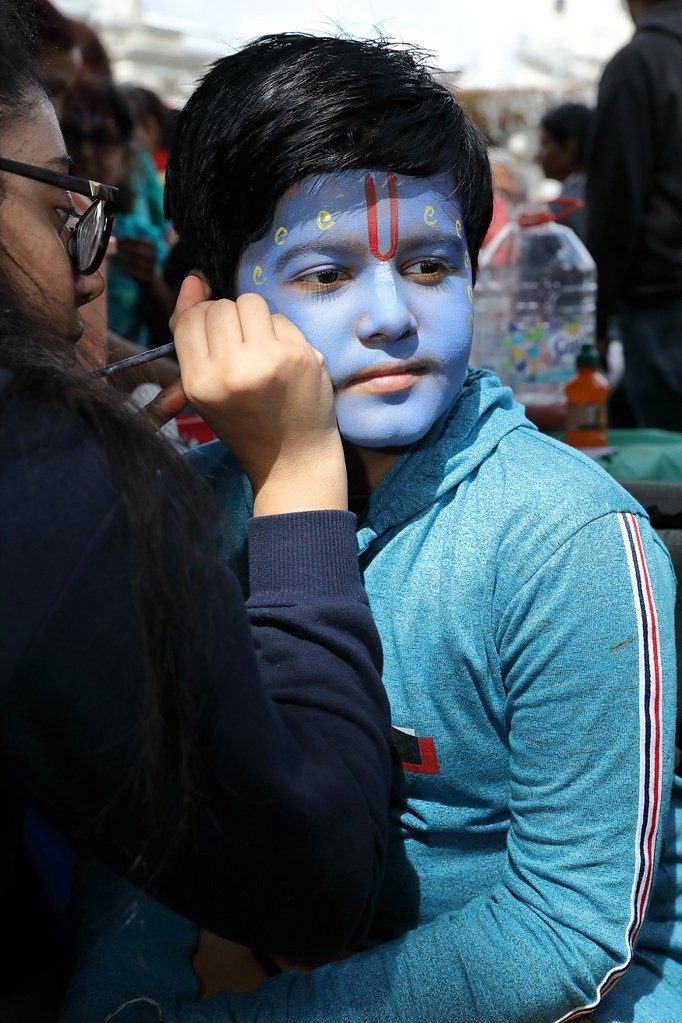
[0,155,121,276]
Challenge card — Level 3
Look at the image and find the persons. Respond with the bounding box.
[0,0,424,1023]
[59,37,682,1023]
[38,16,177,440]
[533,0,682,433]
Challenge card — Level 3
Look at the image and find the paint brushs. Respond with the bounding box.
[89,341,175,380]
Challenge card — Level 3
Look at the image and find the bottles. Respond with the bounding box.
[469,199,598,432]
[564,346,607,447]
